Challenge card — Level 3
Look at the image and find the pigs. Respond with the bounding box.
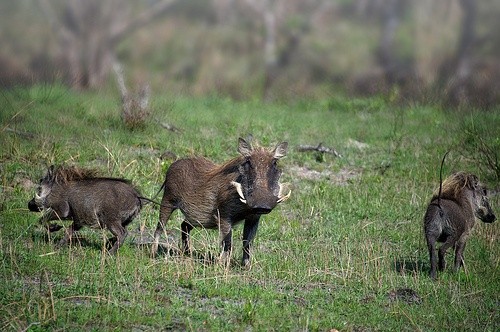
[28,162,181,256]
[144,136,292,270]
[423,150,498,280]
